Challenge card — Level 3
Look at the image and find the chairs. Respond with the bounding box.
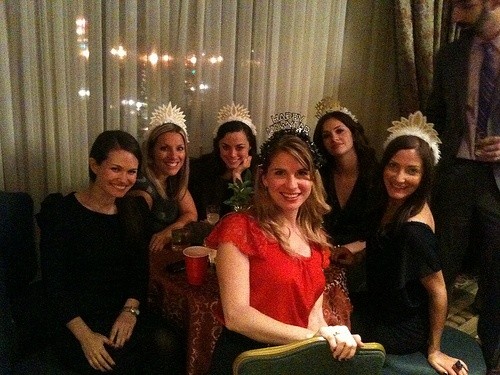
[384,325,488,375]
[0,192,66,375]
[232,335,386,375]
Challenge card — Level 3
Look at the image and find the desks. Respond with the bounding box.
[144,234,353,375]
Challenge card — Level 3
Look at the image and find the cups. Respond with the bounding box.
[183,246,209,285]
[473,132,499,157]
[171,226,191,252]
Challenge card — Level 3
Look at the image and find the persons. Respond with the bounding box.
[426,1,499,375]
[209,130,367,375]
[312,112,380,290]
[332,137,469,375]
[187,121,261,216]
[41,131,181,375]
[139,124,198,253]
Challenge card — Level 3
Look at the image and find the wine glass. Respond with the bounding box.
[206,204,221,230]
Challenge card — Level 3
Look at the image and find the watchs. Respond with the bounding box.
[120,305,141,318]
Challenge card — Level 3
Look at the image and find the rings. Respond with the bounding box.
[451,360,461,372]
[333,331,339,336]
